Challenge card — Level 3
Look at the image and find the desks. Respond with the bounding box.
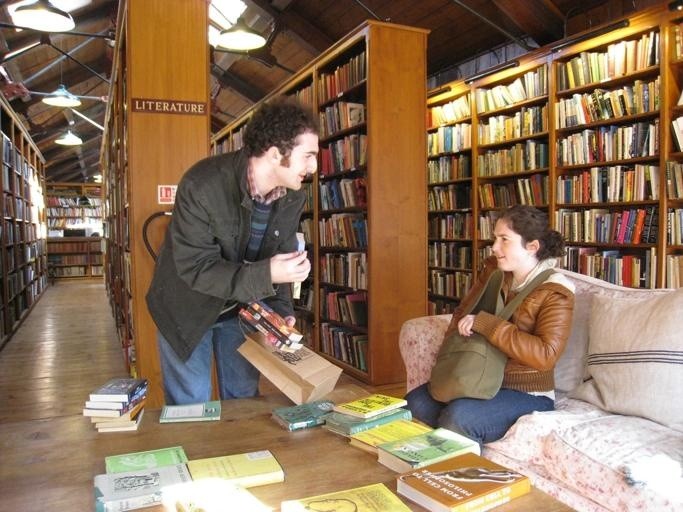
[0,383,580,512]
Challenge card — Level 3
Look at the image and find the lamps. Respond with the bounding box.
[54,129,83,146]
[11,0,76,34]
[40,33,81,107]
[219,17,267,52]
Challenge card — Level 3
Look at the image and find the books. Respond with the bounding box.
[396,451,532,512]
[319,132,368,176]
[104,444,188,474]
[282,481,412,512]
[427,241,471,269]
[427,152,470,184]
[320,99,365,138]
[271,391,482,475]
[319,250,364,288]
[299,281,366,327]
[665,158,683,201]
[427,298,457,315]
[665,253,683,290]
[429,177,455,211]
[427,122,471,155]
[320,321,370,373]
[476,243,494,270]
[668,22,683,60]
[209,122,248,156]
[669,116,683,152]
[429,210,474,240]
[427,94,473,128]
[158,399,223,424]
[0,136,47,340]
[551,117,660,169]
[301,173,366,210]
[474,64,547,114]
[290,232,308,301]
[550,202,659,245]
[47,195,103,278]
[475,211,499,240]
[81,377,146,433]
[478,173,545,209]
[666,206,683,247]
[91,463,193,511]
[477,101,547,144]
[545,163,658,206]
[186,450,285,489]
[557,243,658,292]
[238,298,303,354]
[476,139,547,176]
[292,52,371,105]
[554,76,662,129]
[554,29,663,93]
[297,211,367,251]
[429,270,472,299]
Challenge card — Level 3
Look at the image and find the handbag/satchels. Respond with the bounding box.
[427,268,556,402]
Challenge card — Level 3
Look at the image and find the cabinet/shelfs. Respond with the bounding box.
[211,19,428,386]
[101,1,220,407]
[49,183,101,277]
[0,90,48,349]
[426,1,683,317]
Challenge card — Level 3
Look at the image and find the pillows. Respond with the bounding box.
[572,291,682,429]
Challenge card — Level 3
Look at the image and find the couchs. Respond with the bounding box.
[399,267,682,511]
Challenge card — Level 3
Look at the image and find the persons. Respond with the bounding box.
[401,205,575,458]
[144,99,319,406]
